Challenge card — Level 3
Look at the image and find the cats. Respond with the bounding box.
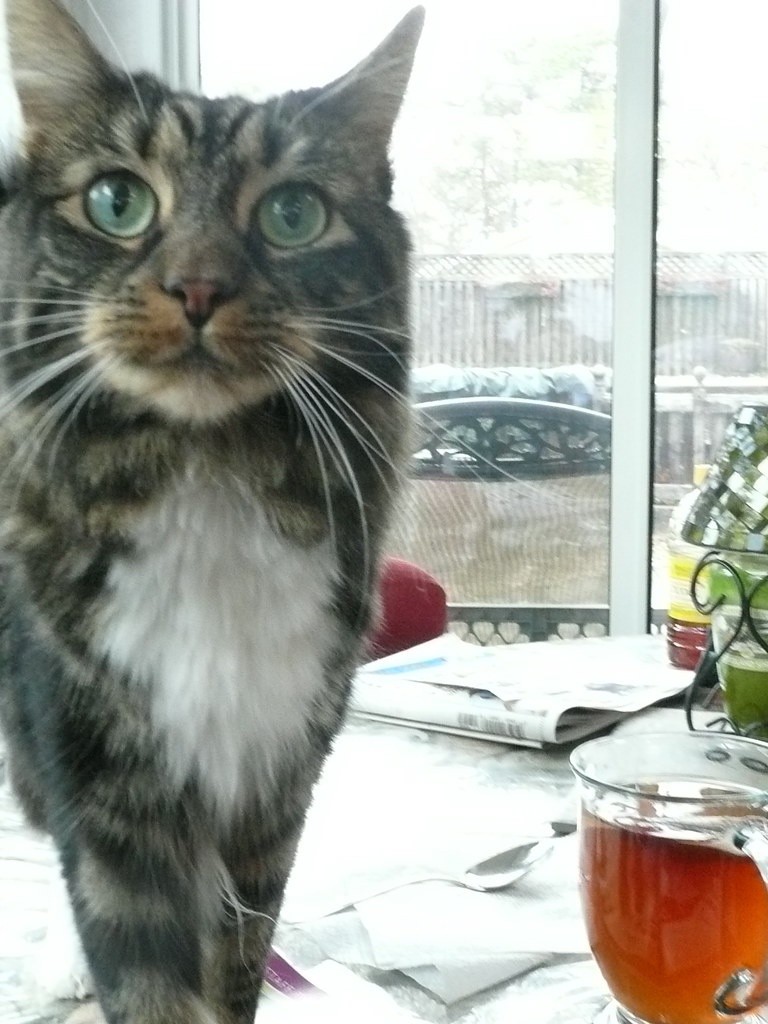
[0,0,429,1023]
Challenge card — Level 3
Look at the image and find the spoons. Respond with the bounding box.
[279,837,554,923]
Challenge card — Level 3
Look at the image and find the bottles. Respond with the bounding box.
[666,463,712,671]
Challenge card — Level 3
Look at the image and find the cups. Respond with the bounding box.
[567,728,768,1024]
